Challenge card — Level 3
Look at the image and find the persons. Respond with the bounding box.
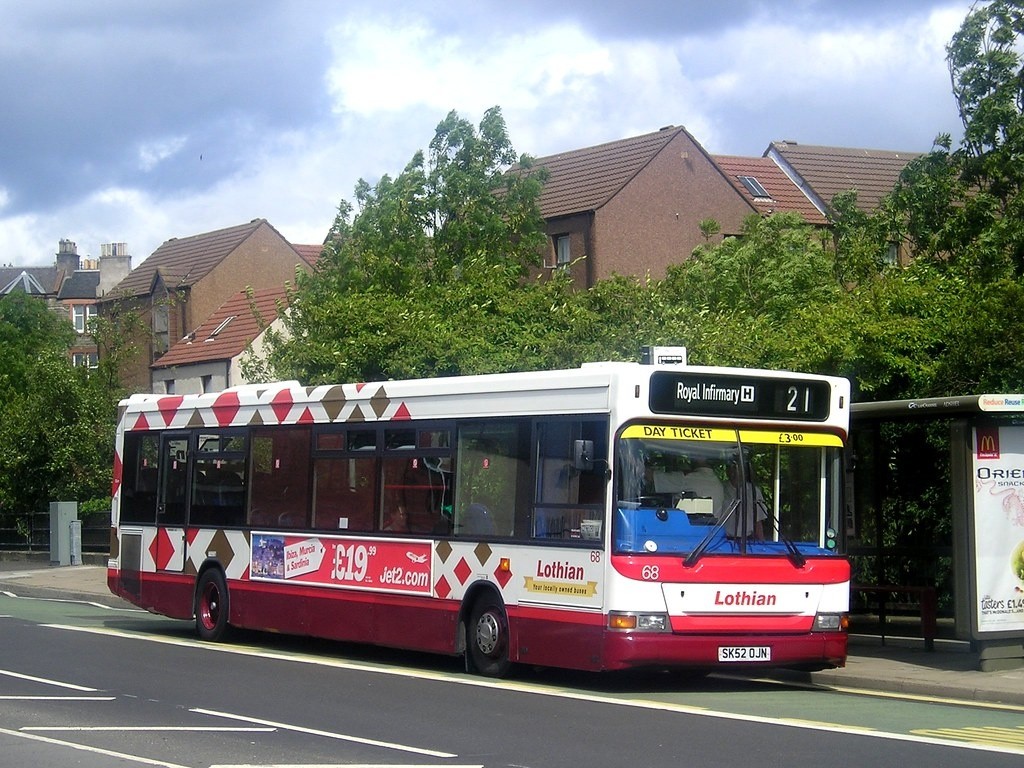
[720,453,768,543]
[384,502,416,534]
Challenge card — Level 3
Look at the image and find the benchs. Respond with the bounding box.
[848,586,939,654]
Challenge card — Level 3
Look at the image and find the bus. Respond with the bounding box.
[107,361,850,679]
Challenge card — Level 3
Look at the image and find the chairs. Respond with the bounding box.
[140,466,441,535]
